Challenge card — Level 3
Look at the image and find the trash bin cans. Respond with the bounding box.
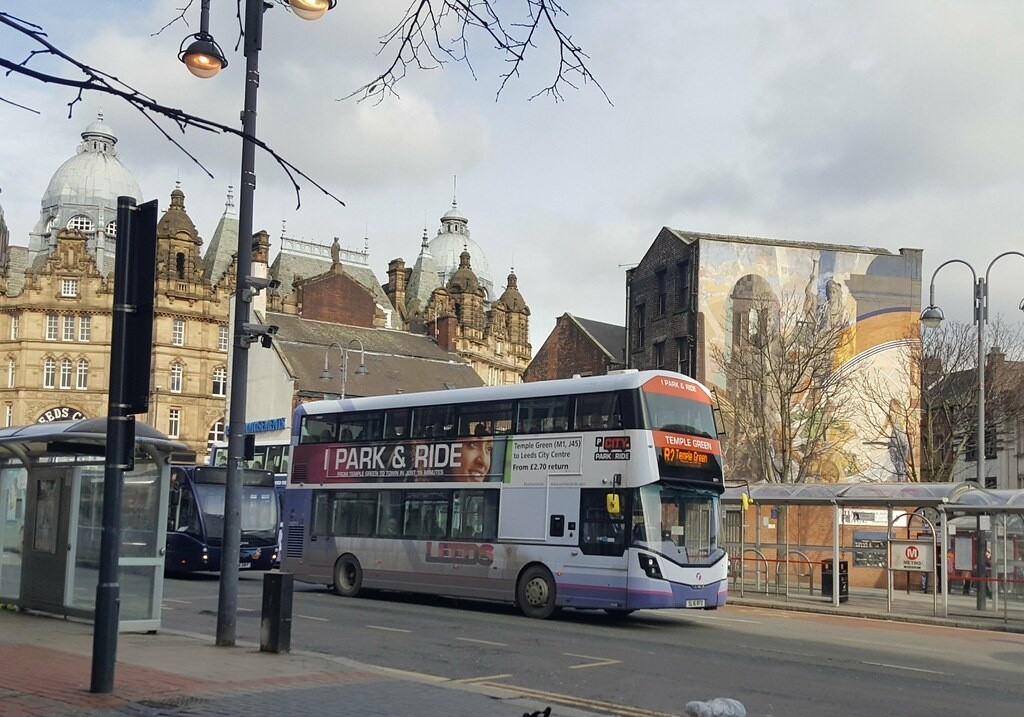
[821,558,849,604]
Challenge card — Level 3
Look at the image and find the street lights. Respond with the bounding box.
[154,384,162,429]
[177,1,330,646]
[918,252,1024,609]
[318,337,370,398]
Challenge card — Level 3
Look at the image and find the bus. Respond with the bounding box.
[78,466,281,574]
[211,440,291,558]
[279,368,755,620]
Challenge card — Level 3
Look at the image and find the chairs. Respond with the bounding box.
[303,412,619,537]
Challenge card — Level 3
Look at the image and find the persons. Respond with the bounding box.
[381,518,399,537]
[319,422,493,442]
[451,434,494,482]
